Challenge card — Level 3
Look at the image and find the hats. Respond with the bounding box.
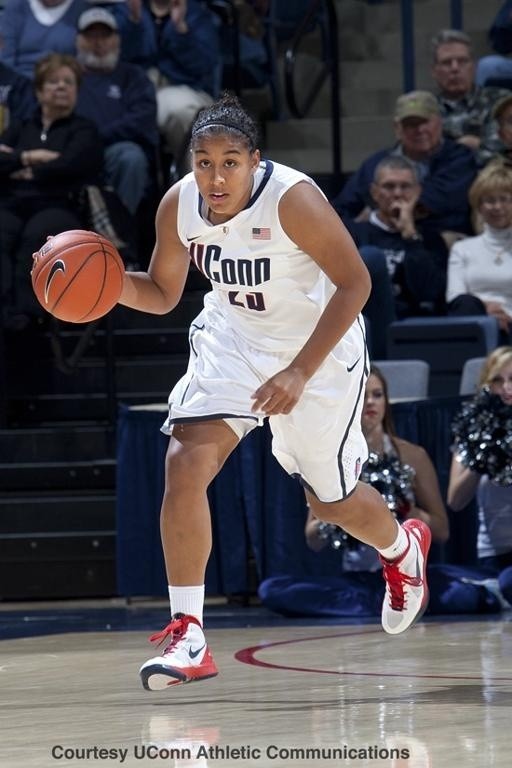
[77,7,120,31]
[395,90,442,123]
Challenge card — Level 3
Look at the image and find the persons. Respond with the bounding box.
[449,343,511,613]
[30,92,433,692]
[0,2,510,351]
[256,361,499,622]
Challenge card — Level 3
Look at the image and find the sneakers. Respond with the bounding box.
[139,613,218,690]
[379,518,433,636]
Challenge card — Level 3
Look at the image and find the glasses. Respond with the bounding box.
[372,181,412,192]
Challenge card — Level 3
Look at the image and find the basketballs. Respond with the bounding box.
[32,229,125,322]
